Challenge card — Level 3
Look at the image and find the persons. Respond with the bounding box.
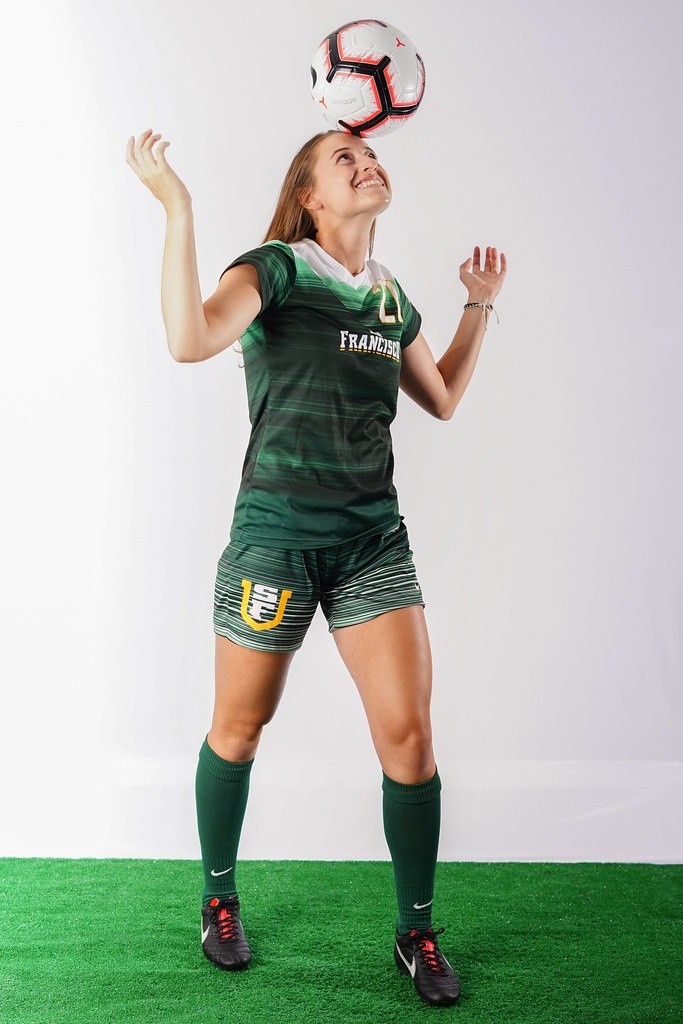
[124,129,506,1007]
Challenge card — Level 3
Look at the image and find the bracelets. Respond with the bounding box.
[463,301,500,331]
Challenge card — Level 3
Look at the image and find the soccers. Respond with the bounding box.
[308,17,432,138]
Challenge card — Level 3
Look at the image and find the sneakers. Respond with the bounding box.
[198,899,249,969]
[393,926,460,1005]
